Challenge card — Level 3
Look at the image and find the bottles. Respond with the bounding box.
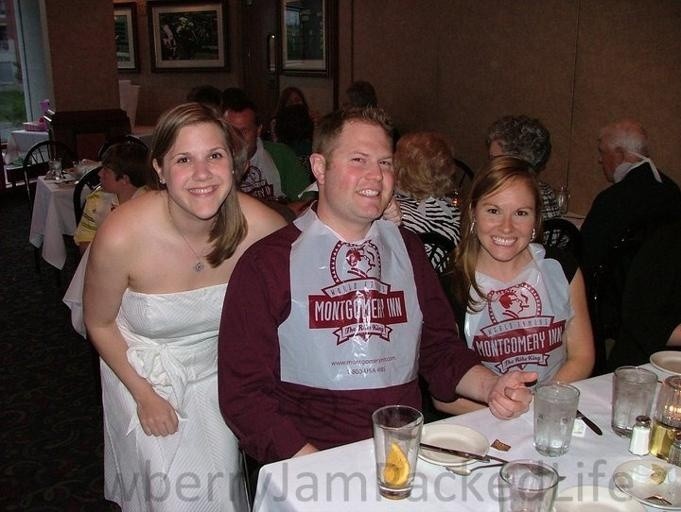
[628,413,654,456]
[648,372,681,462]
[554,185,571,215]
[668,434,680,468]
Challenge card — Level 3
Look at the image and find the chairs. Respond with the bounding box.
[597,234,640,341]
[23,140,76,287]
[418,231,457,277]
[73,166,104,225]
[455,159,474,195]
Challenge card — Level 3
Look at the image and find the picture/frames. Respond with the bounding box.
[111,2,138,72]
[147,0,231,73]
[280,0,333,78]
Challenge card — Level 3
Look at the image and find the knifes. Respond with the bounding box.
[574,408,604,437]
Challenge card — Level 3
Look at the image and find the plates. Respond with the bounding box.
[612,460,680,511]
[412,420,491,469]
[650,350,681,377]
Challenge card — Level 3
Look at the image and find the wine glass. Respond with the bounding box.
[52,157,65,183]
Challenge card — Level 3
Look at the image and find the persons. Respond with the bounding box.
[175,17,201,60]
[76,83,680,510]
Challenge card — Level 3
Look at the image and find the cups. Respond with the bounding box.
[533,381,581,458]
[499,458,559,511]
[370,403,422,502]
[610,365,659,444]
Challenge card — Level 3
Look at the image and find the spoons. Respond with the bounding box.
[444,463,515,477]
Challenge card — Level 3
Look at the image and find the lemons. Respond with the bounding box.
[384,443,411,485]
